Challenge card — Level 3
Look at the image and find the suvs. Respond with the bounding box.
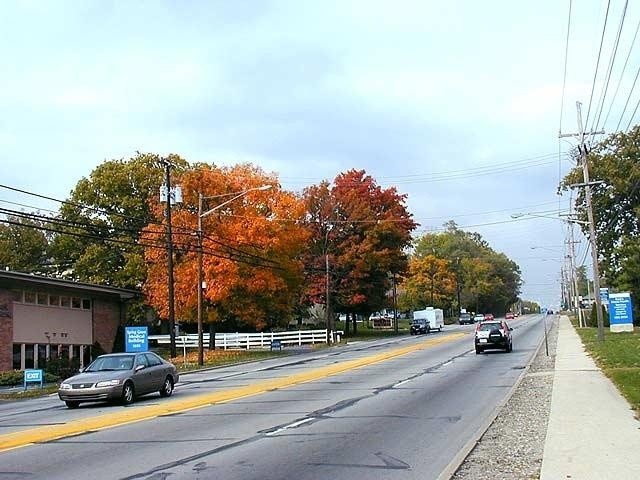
[409,318,434,336]
[475,319,516,355]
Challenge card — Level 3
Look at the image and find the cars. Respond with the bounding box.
[338,312,401,323]
[459,312,520,324]
[547,310,554,315]
[55,351,183,409]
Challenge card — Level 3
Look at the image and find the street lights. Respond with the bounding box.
[542,256,575,312]
[196,183,274,365]
[455,254,463,313]
[530,244,583,328]
[510,211,606,342]
